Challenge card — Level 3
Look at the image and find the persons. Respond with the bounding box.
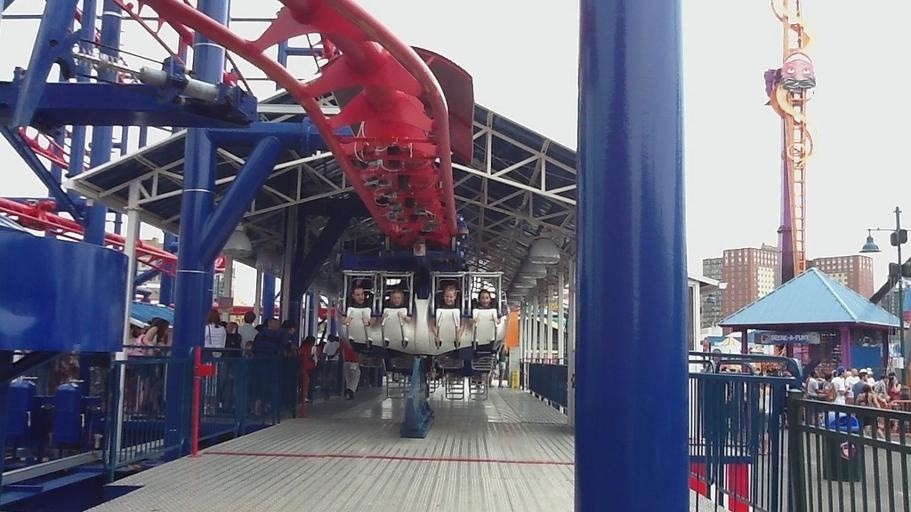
[337,286,378,328]
[468,289,502,329]
[377,290,413,326]
[429,284,467,346]
[804,362,904,440]
[131,304,365,417]
[486,345,511,388]
[48,351,111,414]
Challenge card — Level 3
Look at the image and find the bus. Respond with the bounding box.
[707,323,774,364]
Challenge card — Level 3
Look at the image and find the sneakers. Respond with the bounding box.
[345,389,354,400]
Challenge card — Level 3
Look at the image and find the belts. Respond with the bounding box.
[346,360,358,362]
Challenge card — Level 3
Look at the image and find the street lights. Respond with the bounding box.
[858,207,911,403]
[705,290,726,326]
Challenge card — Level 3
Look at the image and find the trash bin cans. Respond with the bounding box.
[822,413,862,482]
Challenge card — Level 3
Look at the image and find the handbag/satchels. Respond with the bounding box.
[134,333,153,356]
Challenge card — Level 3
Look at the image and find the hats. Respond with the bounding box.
[867,368,873,374]
[835,367,843,377]
